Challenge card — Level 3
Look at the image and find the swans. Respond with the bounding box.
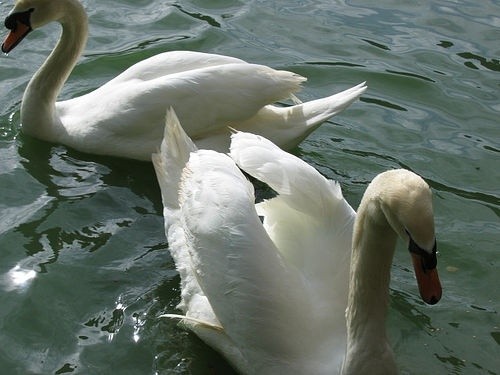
[149,104,444,375]
[0,0,368,168]
